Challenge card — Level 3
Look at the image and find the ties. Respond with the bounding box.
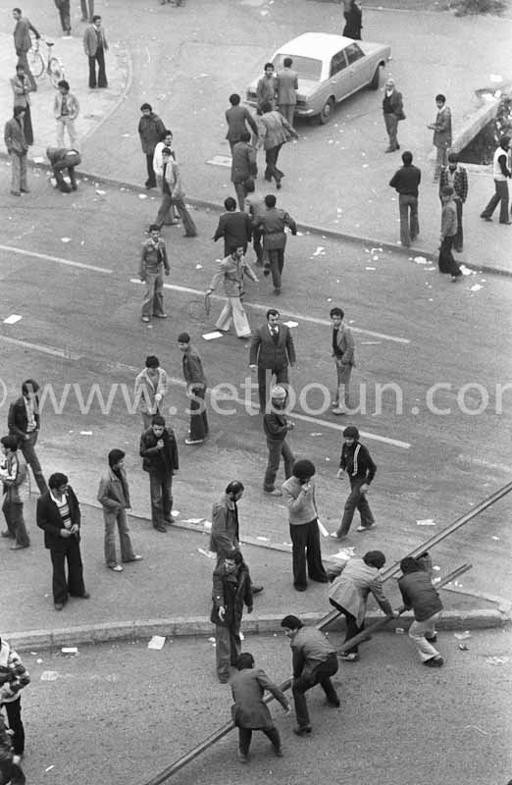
[271,328,278,346]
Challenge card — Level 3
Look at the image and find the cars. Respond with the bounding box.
[243,30,394,126]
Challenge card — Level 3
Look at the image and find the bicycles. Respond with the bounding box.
[26,35,66,91]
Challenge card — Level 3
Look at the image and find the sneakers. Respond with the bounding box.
[272,744,284,756]
[330,531,339,538]
[357,521,376,532]
[264,487,282,497]
[141,312,168,323]
[332,400,345,415]
[237,748,247,763]
[263,259,271,277]
[183,428,205,445]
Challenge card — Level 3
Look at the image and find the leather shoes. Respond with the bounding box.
[422,656,444,667]
[217,671,229,682]
[251,586,263,593]
[11,542,24,550]
[78,591,90,598]
[112,564,125,573]
[167,515,178,525]
[0,530,10,538]
[293,724,313,735]
[130,555,141,561]
[426,634,437,643]
[54,599,64,610]
[159,525,165,534]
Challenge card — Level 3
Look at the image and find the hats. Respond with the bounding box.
[268,385,287,399]
